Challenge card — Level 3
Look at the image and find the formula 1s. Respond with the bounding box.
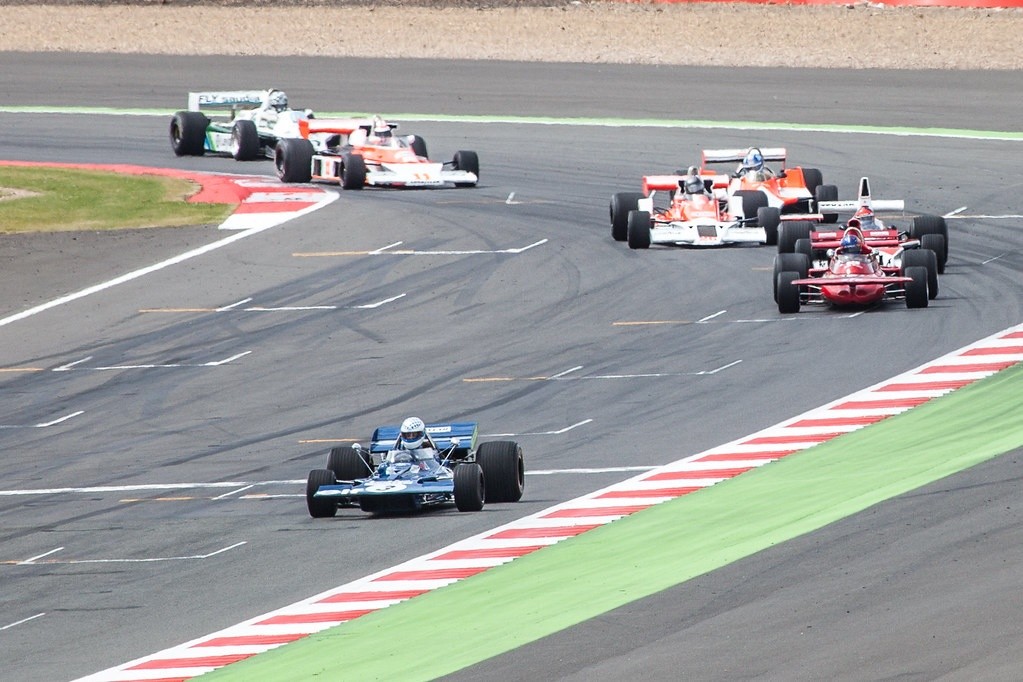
[303,416,533,518]
[273,118,481,194]
[776,179,946,278]
[169,89,322,160]
[771,220,942,310]
[695,148,839,227]
[609,165,778,254]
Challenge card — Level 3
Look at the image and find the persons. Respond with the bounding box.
[268,93,288,111]
[371,125,394,145]
[840,236,862,254]
[855,208,875,223]
[378,416,441,474]
[743,154,764,172]
[685,177,704,194]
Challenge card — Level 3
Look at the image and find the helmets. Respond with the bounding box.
[684,177,705,194]
[270,91,288,106]
[374,120,392,133]
[400,417,426,450]
[857,207,873,217]
[744,154,764,167]
[841,235,859,254]
